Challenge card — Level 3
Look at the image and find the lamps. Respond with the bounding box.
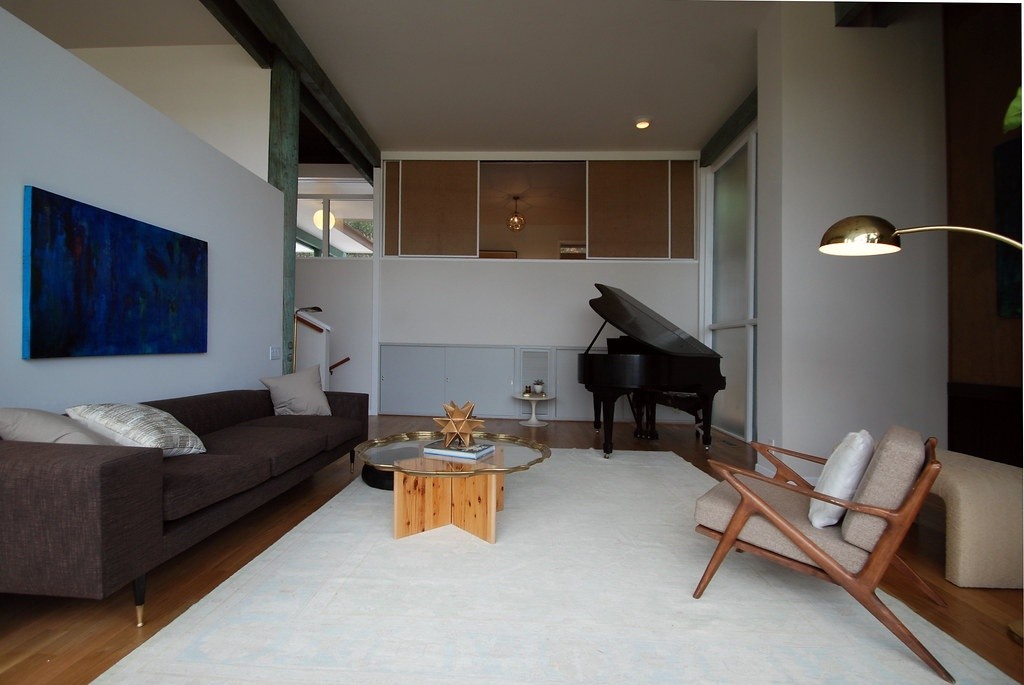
[313,200,335,231]
[818,213,1022,259]
[292,306,323,374]
[505,195,526,233]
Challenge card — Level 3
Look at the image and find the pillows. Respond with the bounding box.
[807,428,878,531]
[261,373,336,416]
[1,406,124,446]
[64,402,210,456]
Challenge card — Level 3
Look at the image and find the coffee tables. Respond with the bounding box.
[354,427,553,544]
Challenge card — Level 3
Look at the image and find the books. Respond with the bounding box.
[423,440,496,460]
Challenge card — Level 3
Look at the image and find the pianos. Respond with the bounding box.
[576,281,727,460]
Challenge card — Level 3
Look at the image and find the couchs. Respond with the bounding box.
[1,388,370,628]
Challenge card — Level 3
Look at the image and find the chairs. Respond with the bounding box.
[689,425,961,684]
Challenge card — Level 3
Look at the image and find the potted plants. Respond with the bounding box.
[533,379,545,394]
